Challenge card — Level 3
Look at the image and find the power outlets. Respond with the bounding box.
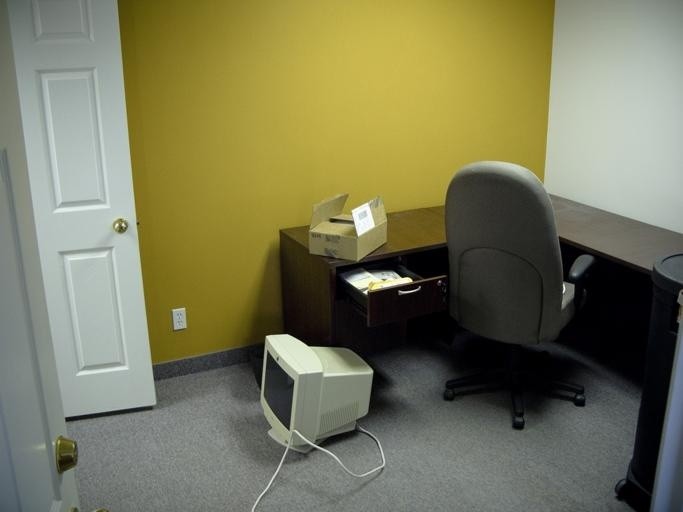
[172,308,187,331]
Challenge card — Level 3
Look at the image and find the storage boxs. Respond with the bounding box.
[309,194,388,261]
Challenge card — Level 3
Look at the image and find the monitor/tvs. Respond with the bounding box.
[260,331,375,455]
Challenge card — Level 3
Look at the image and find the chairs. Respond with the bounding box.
[427,162,595,429]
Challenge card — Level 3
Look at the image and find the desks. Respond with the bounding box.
[279,207,450,357]
[549,194,683,390]
